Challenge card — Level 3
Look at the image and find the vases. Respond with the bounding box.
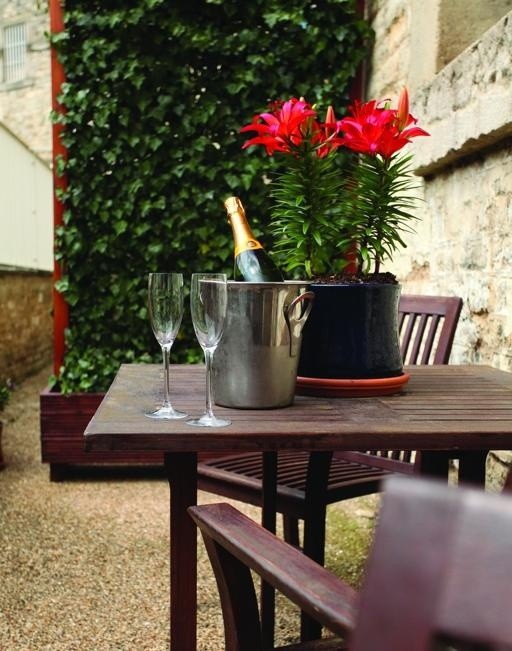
[297,273,410,393]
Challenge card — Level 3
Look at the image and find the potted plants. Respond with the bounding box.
[34,2,365,472]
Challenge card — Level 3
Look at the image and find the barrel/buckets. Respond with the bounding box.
[198,277,316,409]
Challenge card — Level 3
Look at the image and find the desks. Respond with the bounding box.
[81,364,509,650]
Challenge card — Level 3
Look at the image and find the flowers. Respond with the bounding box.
[239,94,434,281]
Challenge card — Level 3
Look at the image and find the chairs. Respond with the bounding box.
[196,291,464,644]
[353,474,512,649]
[191,497,355,651]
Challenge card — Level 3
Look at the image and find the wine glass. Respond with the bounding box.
[185,273,233,428]
[144,273,190,420]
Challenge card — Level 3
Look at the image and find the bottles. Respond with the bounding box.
[224,196,286,282]
